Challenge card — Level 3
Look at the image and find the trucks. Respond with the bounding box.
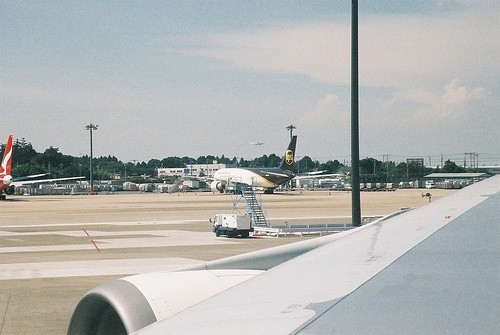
[209,214,251,238]
[332,179,470,193]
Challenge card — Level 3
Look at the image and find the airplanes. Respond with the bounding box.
[182,134,298,194]
[0,135,86,200]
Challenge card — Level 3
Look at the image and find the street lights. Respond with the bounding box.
[85,124,99,192]
[286,124,296,140]
